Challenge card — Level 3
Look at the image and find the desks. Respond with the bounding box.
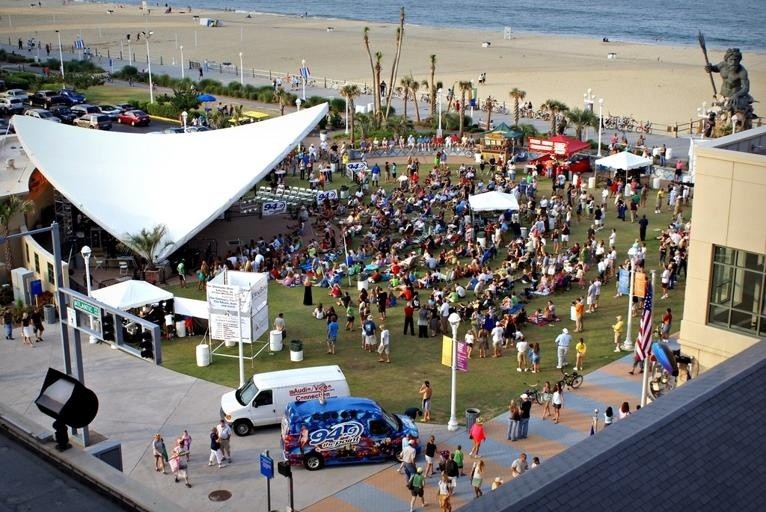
[104,256,139,273]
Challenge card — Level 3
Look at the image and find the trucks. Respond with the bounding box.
[277,390,422,476]
[216,361,356,439]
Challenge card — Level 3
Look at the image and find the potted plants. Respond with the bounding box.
[123,223,176,284]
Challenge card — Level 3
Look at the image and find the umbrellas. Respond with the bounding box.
[196,93,216,110]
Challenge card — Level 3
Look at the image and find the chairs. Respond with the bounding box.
[252,185,323,208]
[93,255,103,271]
[119,261,128,275]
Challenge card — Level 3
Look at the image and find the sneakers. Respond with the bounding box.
[162,471,168,475]
[185,483,191,488]
[517,368,540,373]
[175,478,179,483]
[154,467,159,472]
[208,457,232,469]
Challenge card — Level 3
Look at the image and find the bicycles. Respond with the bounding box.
[519,378,544,405]
[603,110,653,135]
[558,367,585,391]
[464,147,475,159]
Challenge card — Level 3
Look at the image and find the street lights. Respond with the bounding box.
[343,82,350,135]
[618,242,639,352]
[54,27,66,90]
[730,114,738,133]
[595,97,605,157]
[181,110,188,133]
[135,30,154,106]
[238,51,244,84]
[435,86,446,138]
[696,100,711,140]
[300,58,308,102]
[125,31,134,66]
[444,308,463,432]
[294,97,301,157]
[469,79,475,124]
[583,86,596,142]
[179,44,184,79]
[79,244,99,344]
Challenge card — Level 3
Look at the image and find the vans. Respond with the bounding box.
[0,96,23,115]
[70,104,100,115]
[0,80,5,91]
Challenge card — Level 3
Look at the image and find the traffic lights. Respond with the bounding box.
[139,327,153,360]
[101,311,115,343]
[276,462,289,478]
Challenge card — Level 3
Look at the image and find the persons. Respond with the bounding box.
[0,100,717,512]
[705,47,754,124]
[447,88,454,100]
[197,58,208,82]
[478,72,487,85]
[378,80,387,97]
[282,72,300,94]
[136,33,140,41]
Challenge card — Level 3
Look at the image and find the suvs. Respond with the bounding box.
[3,88,27,102]
[116,103,135,110]
[57,87,86,104]
[48,104,75,123]
[26,89,63,108]
[22,107,61,124]
[71,113,112,131]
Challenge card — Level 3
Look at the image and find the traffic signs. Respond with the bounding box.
[71,298,99,319]
[258,453,273,479]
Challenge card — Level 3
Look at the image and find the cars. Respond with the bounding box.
[181,124,210,132]
[97,104,121,119]
[0,128,11,135]
[0,118,13,131]
[160,126,184,134]
[115,109,150,126]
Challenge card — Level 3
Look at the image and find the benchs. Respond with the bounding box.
[350,143,479,157]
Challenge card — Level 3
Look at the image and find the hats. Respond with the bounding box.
[520,394,528,398]
[562,328,569,334]
[417,467,423,473]
[475,416,485,424]
[408,439,415,445]
[616,315,623,319]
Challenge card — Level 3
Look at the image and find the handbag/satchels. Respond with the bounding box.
[406,480,413,490]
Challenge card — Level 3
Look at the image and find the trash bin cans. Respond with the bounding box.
[289,340,304,362]
[465,407,481,433]
